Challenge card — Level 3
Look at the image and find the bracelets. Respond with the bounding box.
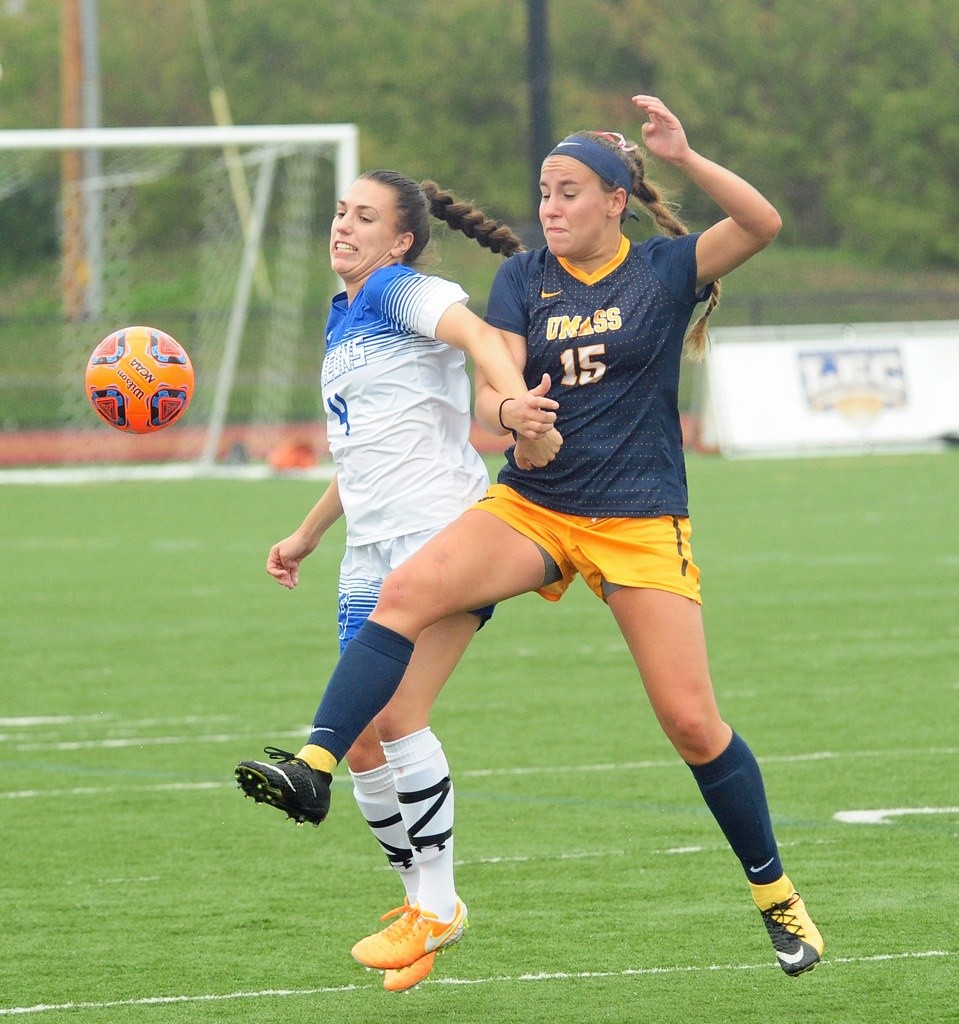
[499,397,516,430]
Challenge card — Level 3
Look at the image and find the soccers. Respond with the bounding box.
[83,324,196,436]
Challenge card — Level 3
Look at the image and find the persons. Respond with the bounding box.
[267,170,562,992]
[238,91,829,978]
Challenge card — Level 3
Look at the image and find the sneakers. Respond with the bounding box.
[233,746,332,827]
[754,890,825,977]
[383,951,436,992]
[351,894,469,972]
